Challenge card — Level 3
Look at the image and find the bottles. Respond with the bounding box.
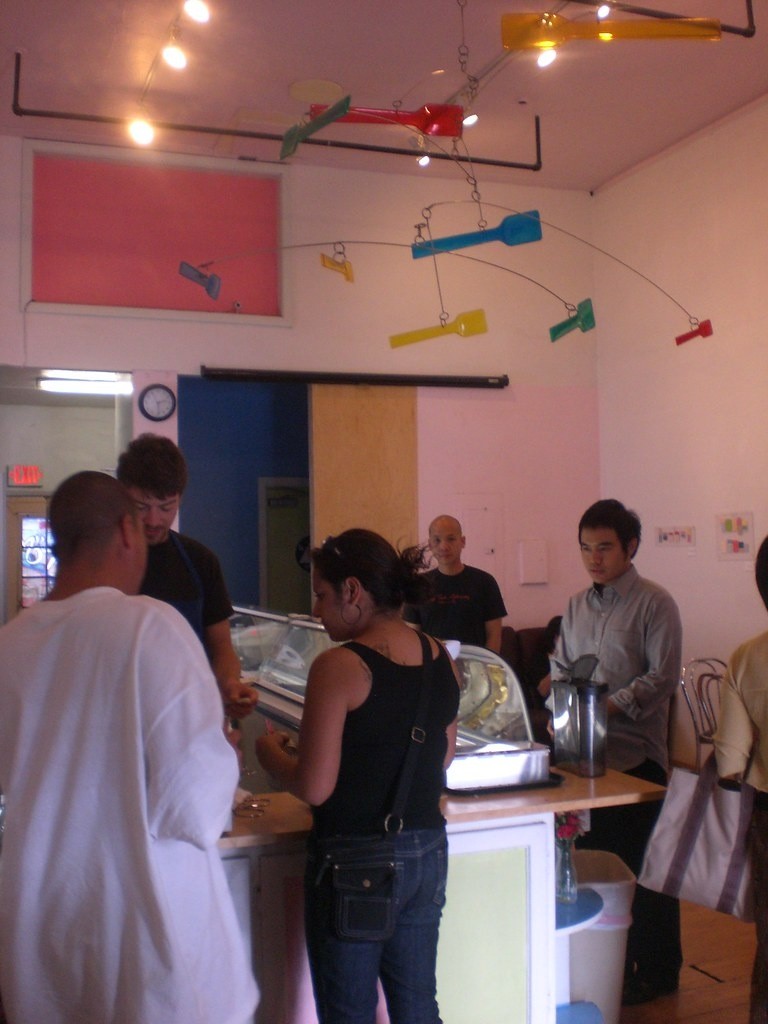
[555,840,578,903]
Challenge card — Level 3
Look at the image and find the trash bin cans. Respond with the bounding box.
[556,850,635,1024]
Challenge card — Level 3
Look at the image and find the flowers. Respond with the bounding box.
[555,810,589,848]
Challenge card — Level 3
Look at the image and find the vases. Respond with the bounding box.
[555,847,578,905]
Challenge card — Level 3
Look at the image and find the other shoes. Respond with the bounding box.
[623,969,681,1006]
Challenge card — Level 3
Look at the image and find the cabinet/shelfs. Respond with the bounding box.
[220,708,668,1024]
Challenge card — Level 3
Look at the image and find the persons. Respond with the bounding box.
[118,435,258,718]
[546,499,681,1000]
[714,533,767,1024]
[401,516,508,654]
[537,615,562,696]
[1,472,260,1024]
[255,528,461,1024]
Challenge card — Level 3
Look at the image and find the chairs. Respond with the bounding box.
[682,658,728,773]
[502,626,546,742]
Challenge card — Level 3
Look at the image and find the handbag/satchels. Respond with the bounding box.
[633,765,767,922]
[324,846,401,945]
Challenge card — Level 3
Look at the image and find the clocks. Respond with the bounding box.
[139,384,176,422]
